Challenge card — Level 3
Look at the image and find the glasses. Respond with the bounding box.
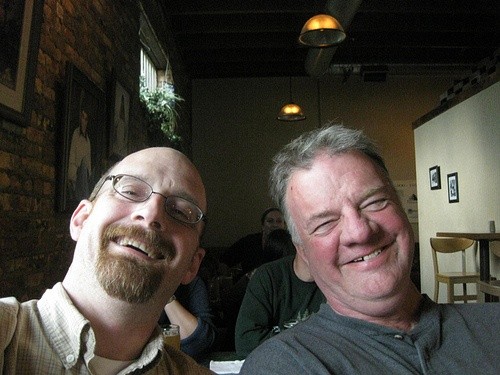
[90,173,207,225]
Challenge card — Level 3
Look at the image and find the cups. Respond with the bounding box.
[162,324,180,350]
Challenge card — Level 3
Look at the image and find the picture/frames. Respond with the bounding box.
[55,59,107,214]
[447,172,459,203]
[0,0,46,128]
[429,165,441,190]
[108,67,133,168]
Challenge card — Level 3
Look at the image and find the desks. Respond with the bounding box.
[436,232,500,302]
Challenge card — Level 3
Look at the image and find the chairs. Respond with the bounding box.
[430,237,480,305]
[477,240,500,304]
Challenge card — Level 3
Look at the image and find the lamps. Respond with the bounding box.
[298,2,347,48]
[277,77,307,121]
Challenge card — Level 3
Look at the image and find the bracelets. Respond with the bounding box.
[165,295,176,306]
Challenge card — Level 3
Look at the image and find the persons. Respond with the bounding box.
[238,125,500,375]
[0,147,218,375]
[157,206,327,357]
